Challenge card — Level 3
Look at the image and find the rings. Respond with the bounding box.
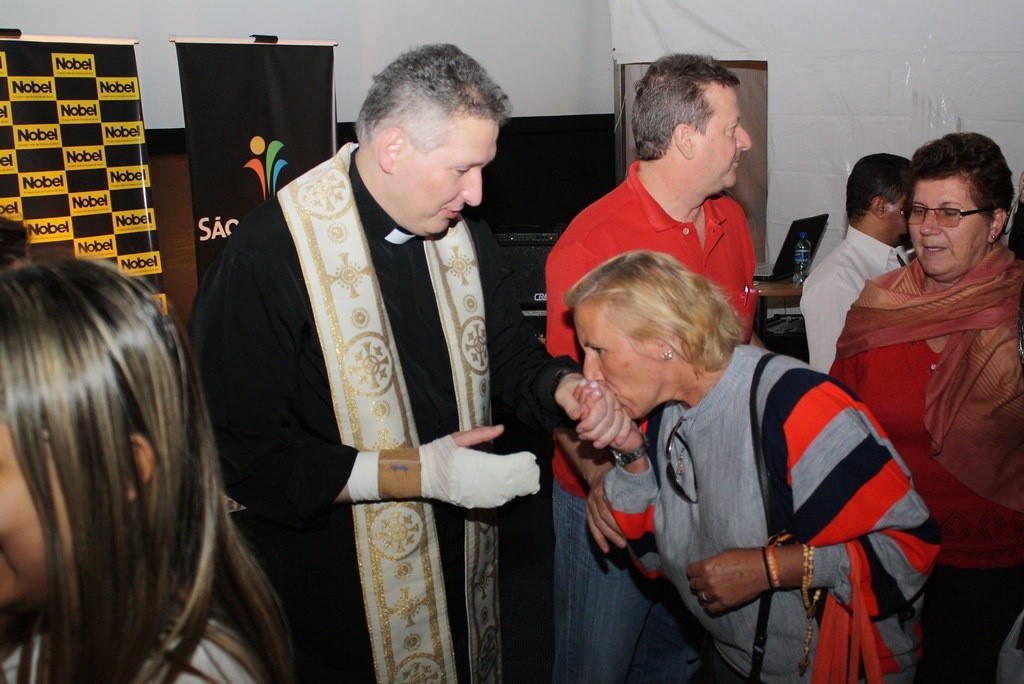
[702,592,707,600]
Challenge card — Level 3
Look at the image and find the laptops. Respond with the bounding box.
[753,214,830,282]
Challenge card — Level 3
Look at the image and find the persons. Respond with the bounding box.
[544,53,766,684]
[0,253,291,684]
[832,129,1024,684]
[1004,169,1024,260]
[798,151,912,375]
[561,249,943,684]
[186,42,634,684]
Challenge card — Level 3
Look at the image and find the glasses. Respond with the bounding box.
[900,203,992,228]
[666,416,699,503]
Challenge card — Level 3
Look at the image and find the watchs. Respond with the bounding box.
[610,433,650,467]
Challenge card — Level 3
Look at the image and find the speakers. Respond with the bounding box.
[493,226,564,339]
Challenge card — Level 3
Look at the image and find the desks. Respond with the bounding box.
[750,281,804,339]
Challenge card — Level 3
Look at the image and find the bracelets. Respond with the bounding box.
[797,543,815,678]
[554,368,579,390]
[762,546,775,589]
[766,546,780,588]
